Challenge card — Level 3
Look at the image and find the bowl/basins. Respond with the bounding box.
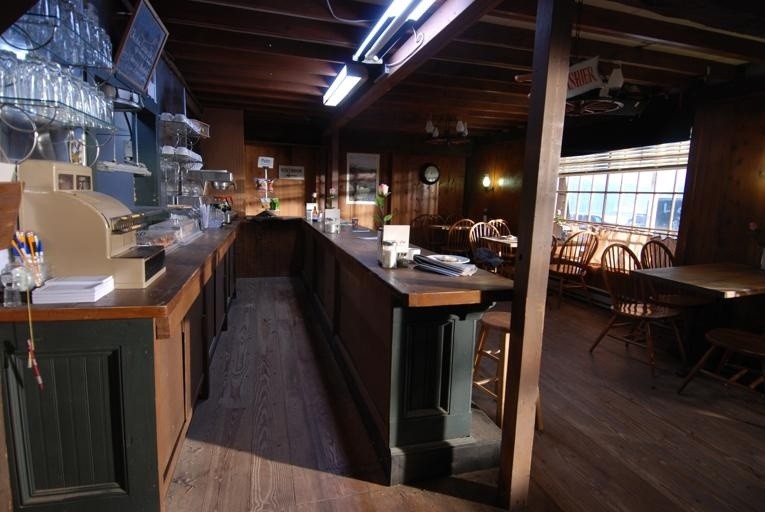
[159,111,204,163]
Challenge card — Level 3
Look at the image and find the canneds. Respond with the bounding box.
[352,219,358,229]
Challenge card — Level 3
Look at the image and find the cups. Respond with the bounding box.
[351,217,359,230]
[0,1,116,128]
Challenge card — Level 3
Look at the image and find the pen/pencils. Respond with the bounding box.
[10,230,43,288]
[27,339,43,390]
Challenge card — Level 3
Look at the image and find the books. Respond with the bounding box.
[30,275,115,306]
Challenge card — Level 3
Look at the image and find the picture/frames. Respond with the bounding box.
[112,0,170,91]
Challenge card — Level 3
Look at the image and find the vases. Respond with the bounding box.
[376,228,383,262]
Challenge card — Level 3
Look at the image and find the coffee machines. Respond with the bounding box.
[185,168,239,222]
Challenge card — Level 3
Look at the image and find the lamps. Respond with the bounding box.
[424,117,469,138]
[322,67,369,109]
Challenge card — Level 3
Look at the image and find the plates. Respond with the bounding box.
[426,253,471,266]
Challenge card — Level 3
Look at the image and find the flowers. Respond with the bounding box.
[326,186,337,208]
[372,182,393,226]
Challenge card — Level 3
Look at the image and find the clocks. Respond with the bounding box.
[421,163,441,184]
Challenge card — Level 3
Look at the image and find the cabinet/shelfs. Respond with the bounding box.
[157,117,207,166]
[234,220,302,277]
[0,12,124,170]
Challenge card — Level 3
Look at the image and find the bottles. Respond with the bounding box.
[396,240,409,268]
[1,269,24,308]
[312,206,323,223]
[212,196,234,225]
[328,217,339,235]
[380,240,397,270]
